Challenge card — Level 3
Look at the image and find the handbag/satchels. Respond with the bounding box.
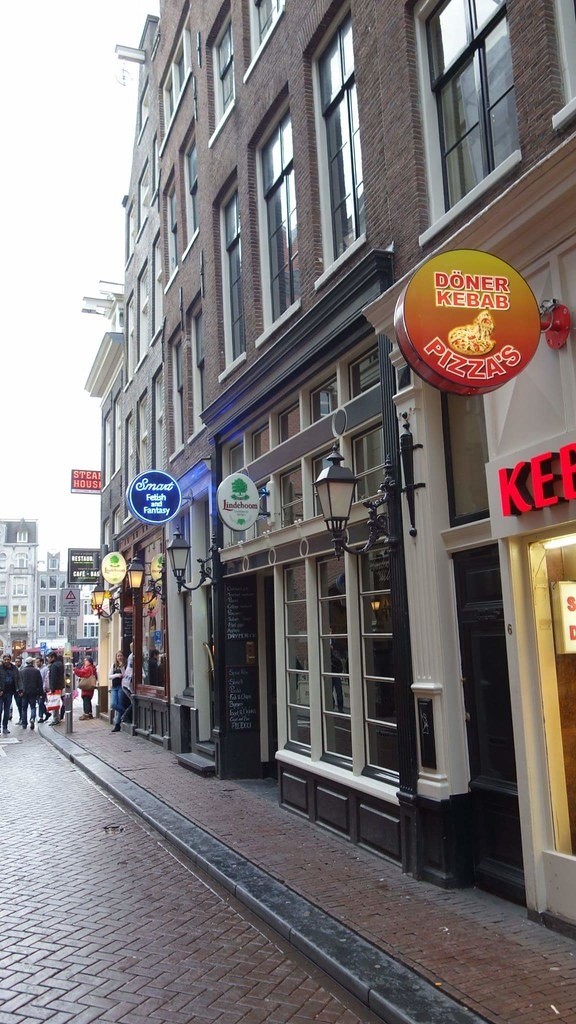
[44,689,63,711]
[77,666,96,690]
[43,671,50,691]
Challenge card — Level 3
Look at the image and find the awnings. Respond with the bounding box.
[25,647,99,653]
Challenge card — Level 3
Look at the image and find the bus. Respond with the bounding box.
[22,645,99,670]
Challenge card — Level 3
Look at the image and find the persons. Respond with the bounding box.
[121,642,150,724]
[330,646,349,712]
[109,650,128,732]
[148,649,167,688]
[296,656,303,691]
[0,652,98,734]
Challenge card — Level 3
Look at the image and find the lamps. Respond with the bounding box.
[91,577,122,618]
[164,522,214,592]
[124,552,166,608]
[313,442,400,561]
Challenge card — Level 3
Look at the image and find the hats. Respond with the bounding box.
[25,657,36,663]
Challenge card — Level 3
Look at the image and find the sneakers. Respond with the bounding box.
[79,714,93,720]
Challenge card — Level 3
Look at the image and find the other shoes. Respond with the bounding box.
[30,719,34,729]
[20,722,28,724]
[121,716,128,721]
[0,730,1,733]
[3,728,10,733]
[44,713,52,721]
[10,709,13,720]
[112,724,121,732]
[49,720,61,726]
[22,724,26,729]
[16,720,21,725]
[38,719,44,723]
